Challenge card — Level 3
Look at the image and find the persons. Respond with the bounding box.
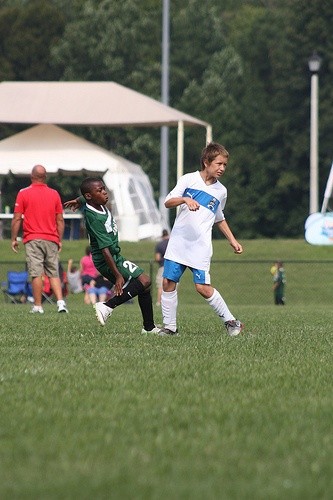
[271,260,288,306]
[153,228,181,305]
[9,164,69,316]
[64,175,169,338]
[152,142,247,338]
[32,245,134,307]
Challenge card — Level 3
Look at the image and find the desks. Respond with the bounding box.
[0,213,85,240]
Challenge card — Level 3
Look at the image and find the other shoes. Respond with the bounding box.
[157,328,178,336]
[29,305,43,314]
[56,300,69,313]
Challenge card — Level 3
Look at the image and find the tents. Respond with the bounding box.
[1,78,218,245]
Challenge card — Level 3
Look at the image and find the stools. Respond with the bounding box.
[27,282,65,305]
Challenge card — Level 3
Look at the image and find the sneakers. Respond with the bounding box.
[224,319,244,337]
[140,324,160,334]
[92,302,114,327]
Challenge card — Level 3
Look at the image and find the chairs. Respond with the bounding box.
[2,271,29,304]
